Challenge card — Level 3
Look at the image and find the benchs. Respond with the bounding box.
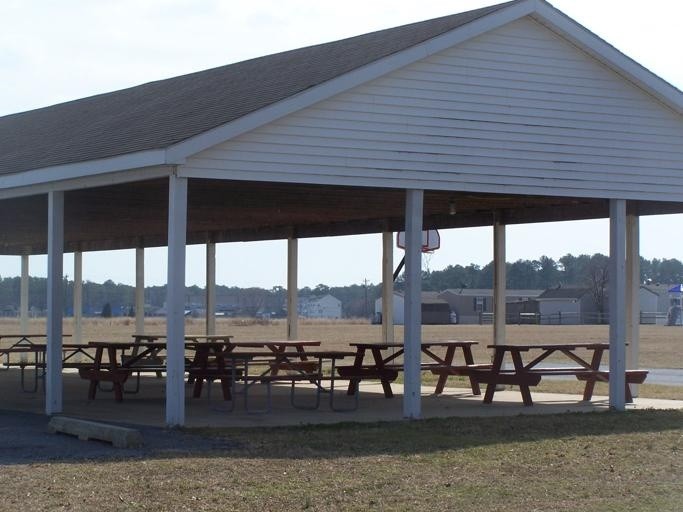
[0,329,649,412]
